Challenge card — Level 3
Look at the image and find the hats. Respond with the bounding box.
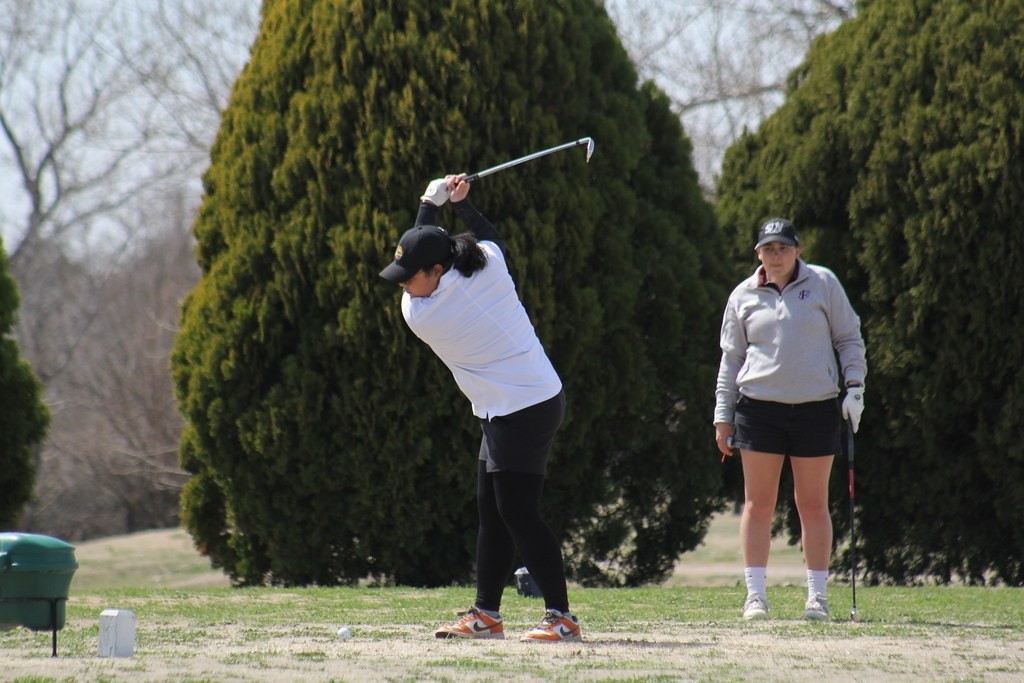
[379,225,451,282]
[755,216,800,248]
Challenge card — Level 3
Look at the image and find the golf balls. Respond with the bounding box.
[336,628,351,640]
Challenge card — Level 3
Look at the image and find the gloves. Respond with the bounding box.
[844,386,862,434]
[420,178,453,206]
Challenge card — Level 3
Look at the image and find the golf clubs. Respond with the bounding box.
[420,137,595,202]
[844,414,862,623]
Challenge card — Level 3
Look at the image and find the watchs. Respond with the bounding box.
[848,383,863,388]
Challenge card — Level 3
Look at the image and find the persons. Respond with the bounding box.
[713,216,867,622]
[378,171,583,641]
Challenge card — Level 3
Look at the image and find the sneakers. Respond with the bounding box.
[435,610,505,639]
[806,598,829,620]
[521,611,580,642]
[742,593,767,620]
